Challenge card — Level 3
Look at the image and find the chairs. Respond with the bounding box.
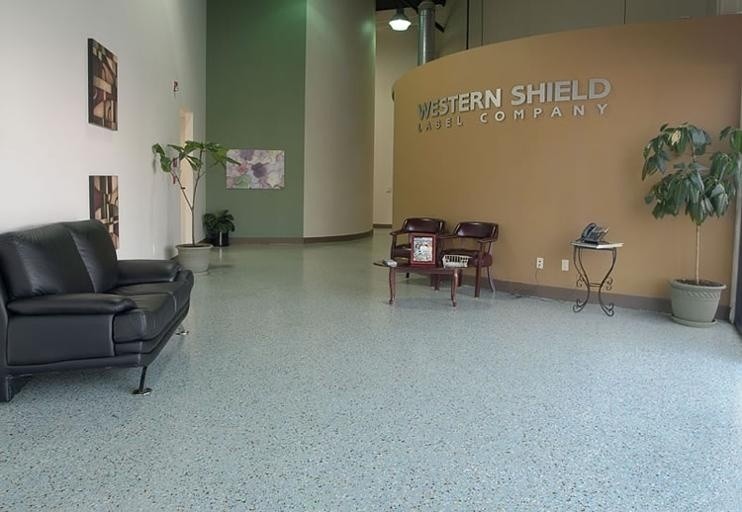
[391,217,442,287]
[440,221,499,298]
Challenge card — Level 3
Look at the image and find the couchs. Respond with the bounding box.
[1,221,195,402]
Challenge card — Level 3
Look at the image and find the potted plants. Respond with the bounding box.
[202,210,235,246]
[641,119,742,329]
[151,140,230,279]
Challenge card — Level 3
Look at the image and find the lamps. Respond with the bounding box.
[387,3,412,32]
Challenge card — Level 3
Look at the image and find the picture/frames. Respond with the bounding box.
[225,149,286,190]
[87,39,121,132]
[407,233,438,266]
[88,174,122,251]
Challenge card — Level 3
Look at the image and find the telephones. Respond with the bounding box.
[582,223,609,243]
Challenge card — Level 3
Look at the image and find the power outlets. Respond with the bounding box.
[537,258,544,269]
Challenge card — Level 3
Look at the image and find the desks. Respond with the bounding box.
[373,252,463,308]
[568,237,624,317]
